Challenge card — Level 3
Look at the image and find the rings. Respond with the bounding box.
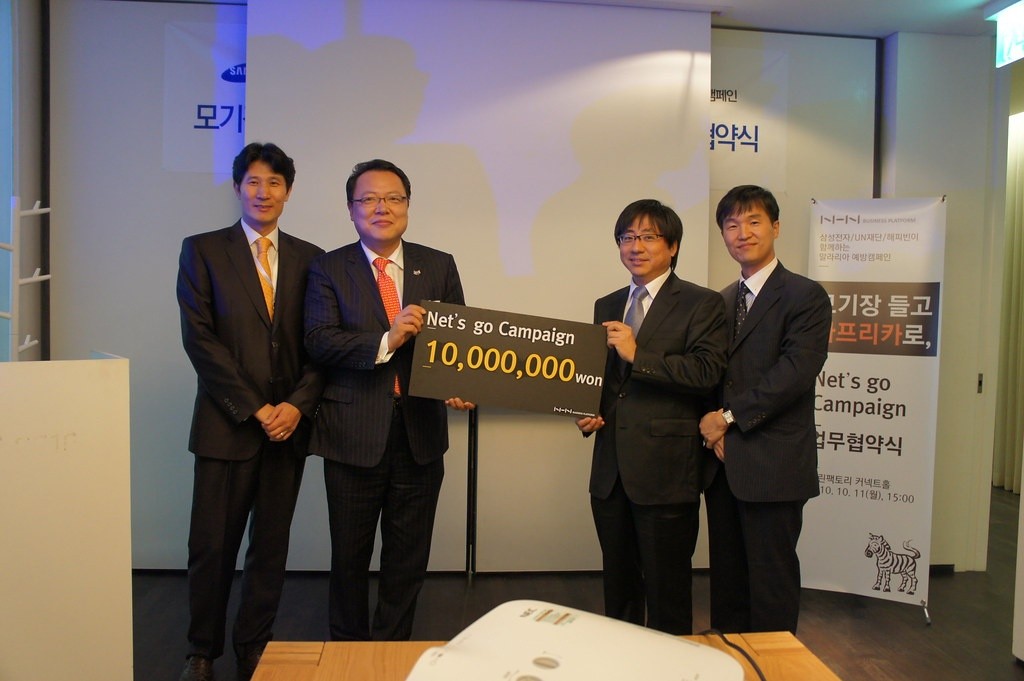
[280,432,283,438]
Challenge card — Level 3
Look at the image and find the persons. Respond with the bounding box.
[306,158,474,646]
[577,199,730,637]
[700,185,832,635]
[176,142,328,681]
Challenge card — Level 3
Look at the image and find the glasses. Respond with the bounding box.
[618,233,664,245]
[352,195,408,208]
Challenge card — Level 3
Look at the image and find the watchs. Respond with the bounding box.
[721,405,736,424]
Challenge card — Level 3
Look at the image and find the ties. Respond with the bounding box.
[616,285,649,380]
[734,281,751,342]
[372,258,403,396]
[255,237,274,324]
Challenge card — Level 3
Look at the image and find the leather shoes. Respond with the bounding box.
[236,646,264,681]
[178,654,215,681]
